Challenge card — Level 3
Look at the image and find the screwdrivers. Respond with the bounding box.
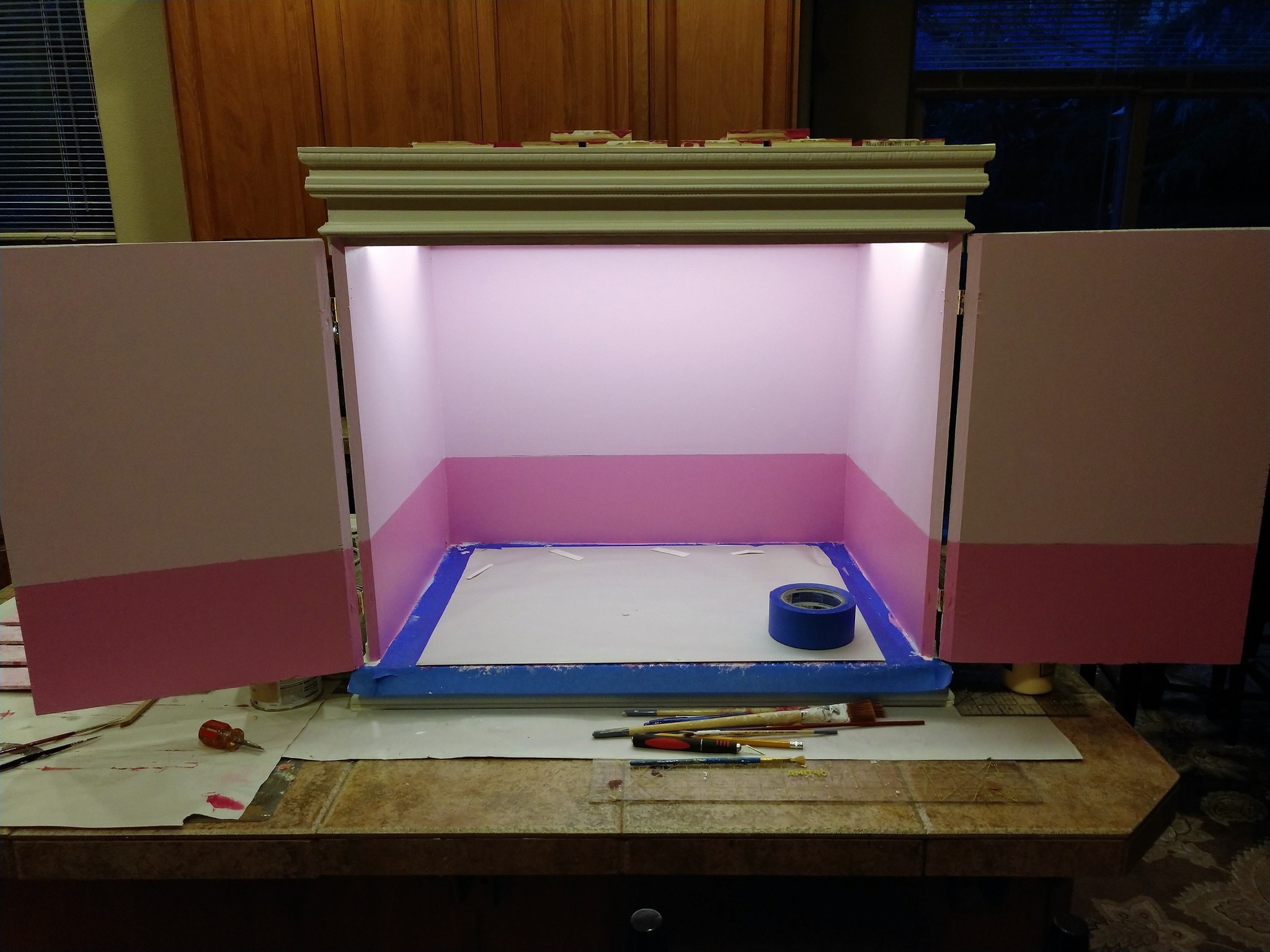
[197,719,265,753]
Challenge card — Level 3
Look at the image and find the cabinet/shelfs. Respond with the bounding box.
[157,0,799,246]
[2,144,1270,719]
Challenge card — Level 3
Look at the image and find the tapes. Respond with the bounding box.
[768,582,857,651]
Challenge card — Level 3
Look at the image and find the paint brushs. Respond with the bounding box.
[0,734,105,773]
[591,697,926,768]
[0,716,124,754]
